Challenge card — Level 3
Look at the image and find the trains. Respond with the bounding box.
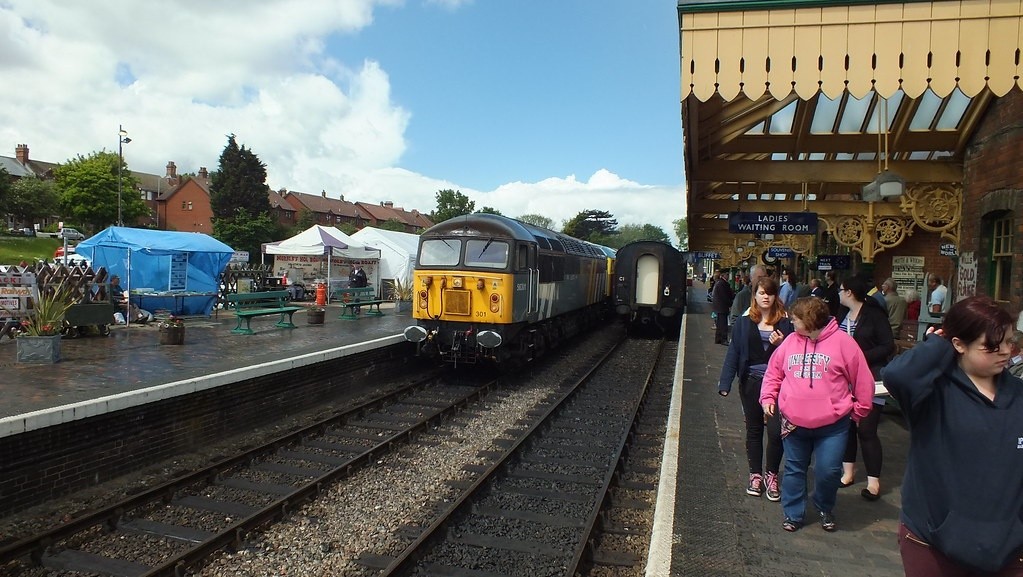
[612,240,688,335]
[404,214,617,381]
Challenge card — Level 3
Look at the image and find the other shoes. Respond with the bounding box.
[860,489,881,499]
[839,478,854,489]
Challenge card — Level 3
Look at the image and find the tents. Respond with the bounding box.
[73,224,236,320]
[260,224,381,306]
[347,226,421,301]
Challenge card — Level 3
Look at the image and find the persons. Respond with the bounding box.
[757,295,876,532]
[281,270,303,302]
[837,276,897,502]
[707,264,948,346]
[717,276,796,502]
[701,272,706,284]
[347,261,366,315]
[879,294,1023,577]
[110,274,149,325]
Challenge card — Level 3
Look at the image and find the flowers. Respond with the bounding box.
[157,306,187,328]
[305,302,325,312]
[0,272,86,337]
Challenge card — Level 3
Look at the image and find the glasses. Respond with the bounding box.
[837,288,845,292]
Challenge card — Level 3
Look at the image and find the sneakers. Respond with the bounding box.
[746,473,764,496]
[764,471,782,501]
[817,511,836,531]
[782,517,802,531]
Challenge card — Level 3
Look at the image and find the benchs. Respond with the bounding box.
[334,287,385,320]
[226,290,300,335]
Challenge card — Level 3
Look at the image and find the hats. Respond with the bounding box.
[110,274,121,281]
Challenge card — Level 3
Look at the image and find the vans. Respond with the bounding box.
[55,228,85,241]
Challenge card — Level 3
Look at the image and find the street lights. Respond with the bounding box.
[118,125,127,226]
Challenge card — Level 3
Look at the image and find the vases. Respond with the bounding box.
[16,334,62,365]
[307,311,325,324]
[159,327,184,345]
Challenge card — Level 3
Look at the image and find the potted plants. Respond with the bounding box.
[388,276,413,313]
